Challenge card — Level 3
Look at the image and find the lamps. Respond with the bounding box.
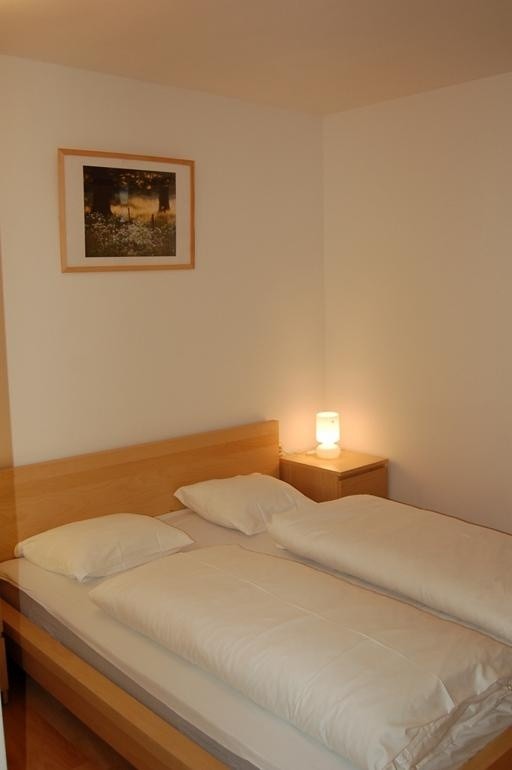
[313,409,343,459]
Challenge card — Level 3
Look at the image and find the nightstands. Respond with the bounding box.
[276,446,389,514]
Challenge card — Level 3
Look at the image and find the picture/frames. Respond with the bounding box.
[55,145,200,274]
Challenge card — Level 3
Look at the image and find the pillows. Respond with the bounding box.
[14,514,194,582]
[173,472,316,536]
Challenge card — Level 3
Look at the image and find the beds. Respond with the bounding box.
[0,418,511,770]
